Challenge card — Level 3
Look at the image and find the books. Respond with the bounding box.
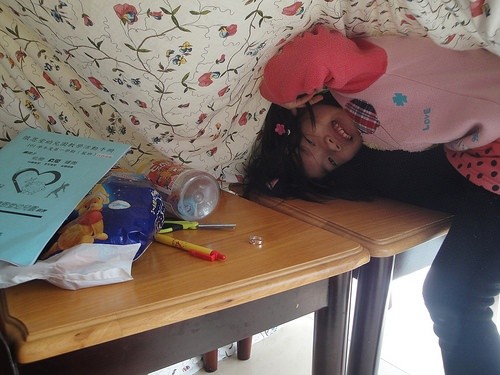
[0,127,131,267]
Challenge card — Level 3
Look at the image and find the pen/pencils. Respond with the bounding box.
[153,233,226,262]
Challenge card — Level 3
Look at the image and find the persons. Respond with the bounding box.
[239,23,500,375]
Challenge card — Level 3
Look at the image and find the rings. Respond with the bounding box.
[249,236,263,244]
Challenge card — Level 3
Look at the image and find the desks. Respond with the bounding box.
[227,183,455,375]
[0,190,371,375]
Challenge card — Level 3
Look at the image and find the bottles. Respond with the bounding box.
[135,153,220,222]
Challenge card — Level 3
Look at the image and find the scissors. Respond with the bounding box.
[159,221,238,233]
